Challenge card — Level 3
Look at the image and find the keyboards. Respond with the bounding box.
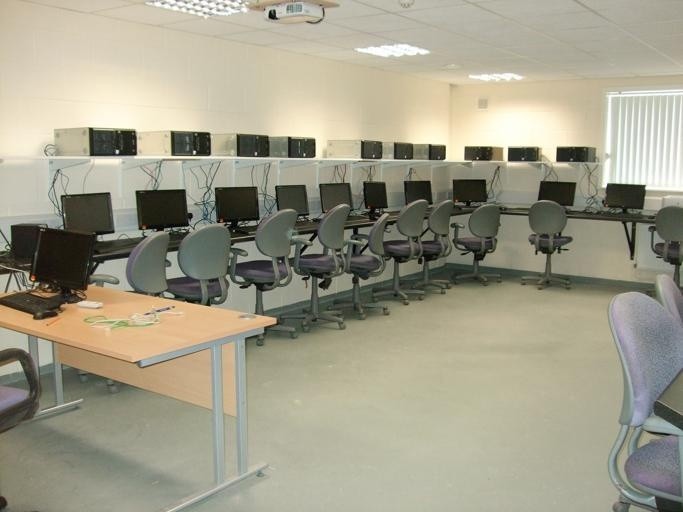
[92,237,141,253]
[0,291,60,315]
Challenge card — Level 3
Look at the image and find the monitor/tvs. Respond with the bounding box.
[60,192,115,242]
[363,181,388,221]
[606,182,646,217]
[135,189,189,231]
[215,186,260,232]
[452,179,487,208]
[319,183,354,213]
[537,181,578,206]
[275,185,310,222]
[29,227,96,304]
[404,181,433,206]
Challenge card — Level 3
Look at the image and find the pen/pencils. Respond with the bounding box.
[46,317,63,326]
[143,305,175,316]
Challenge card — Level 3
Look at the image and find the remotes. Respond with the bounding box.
[78,300,103,308]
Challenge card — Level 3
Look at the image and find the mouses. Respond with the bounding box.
[499,205,508,211]
[648,215,656,219]
[33,311,58,320]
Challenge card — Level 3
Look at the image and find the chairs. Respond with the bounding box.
[76,231,169,394]
[164,224,230,308]
[404,200,452,294]
[653,273,682,326]
[647,205,682,289]
[226,208,298,347]
[605,291,683,512]
[371,199,428,305]
[0,348,42,509]
[323,213,391,319]
[448,204,502,286]
[520,200,573,290]
[276,203,350,332]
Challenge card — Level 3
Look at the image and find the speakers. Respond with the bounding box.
[11,223,47,262]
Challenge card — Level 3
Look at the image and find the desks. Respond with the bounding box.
[0,284,277,512]
[0,204,658,282]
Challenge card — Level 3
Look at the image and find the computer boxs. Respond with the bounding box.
[413,144,446,161]
[54,127,138,157]
[508,147,542,161]
[384,142,413,160]
[211,134,269,157]
[556,147,596,162]
[327,140,383,159]
[137,131,212,157]
[464,146,504,162]
[269,137,316,158]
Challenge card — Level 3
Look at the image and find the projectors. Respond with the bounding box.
[266,2,323,20]
